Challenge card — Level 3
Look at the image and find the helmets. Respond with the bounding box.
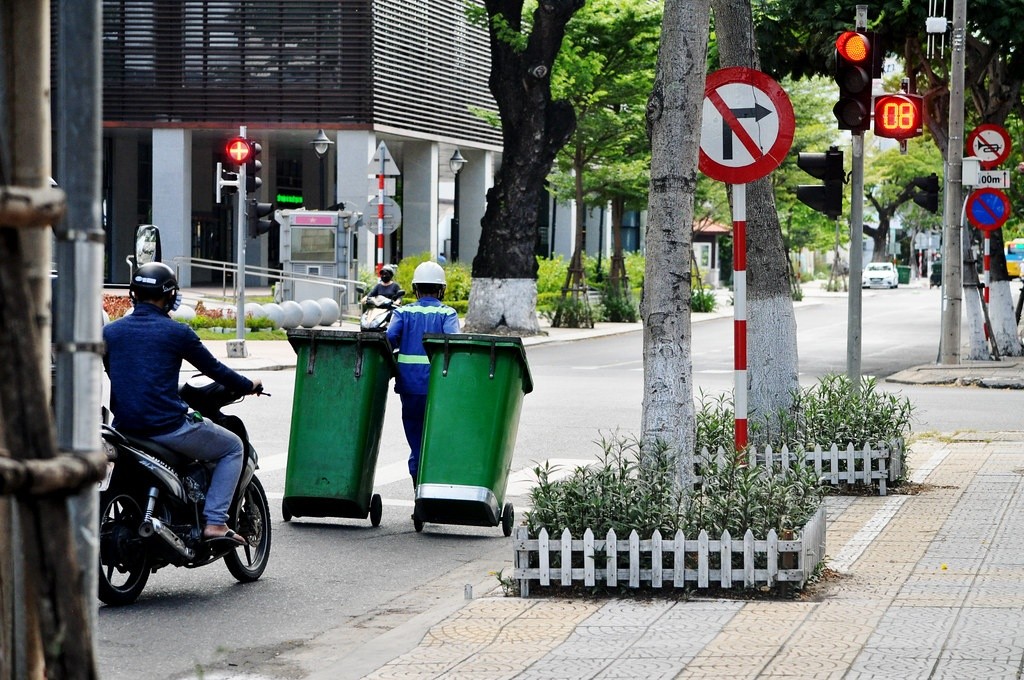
[412,261,445,285]
[380,265,394,281]
[132,262,179,286]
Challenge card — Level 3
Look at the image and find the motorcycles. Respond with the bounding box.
[933,271,942,288]
[95,370,274,605]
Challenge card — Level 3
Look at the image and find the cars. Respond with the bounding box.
[859,260,902,291]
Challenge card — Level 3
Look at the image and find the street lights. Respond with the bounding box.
[449,149,469,264]
[309,128,335,211]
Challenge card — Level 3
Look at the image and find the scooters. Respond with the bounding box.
[355,286,406,332]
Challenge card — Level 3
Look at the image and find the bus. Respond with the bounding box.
[1004,237,1024,282]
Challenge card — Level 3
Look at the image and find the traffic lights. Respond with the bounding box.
[873,91,923,140]
[246,141,264,192]
[832,30,874,136]
[796,147,842,221]
[907,172,941,214]
[228,137,250,167]
[248,198,273,238]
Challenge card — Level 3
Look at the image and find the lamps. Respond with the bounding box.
[307,130,335,157]
[449,146,467,174]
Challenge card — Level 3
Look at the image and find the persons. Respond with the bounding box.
[439,252,446,265]
[103,262,263,545]
[932,254,942,274]
[360,265,402,305]
[386,262,460,493]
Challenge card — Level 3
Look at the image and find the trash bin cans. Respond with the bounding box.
[411,332,534,537]
[281,328,397,526]
[897,265,911,284]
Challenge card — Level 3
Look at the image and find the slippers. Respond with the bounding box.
[204,529,248,546]
